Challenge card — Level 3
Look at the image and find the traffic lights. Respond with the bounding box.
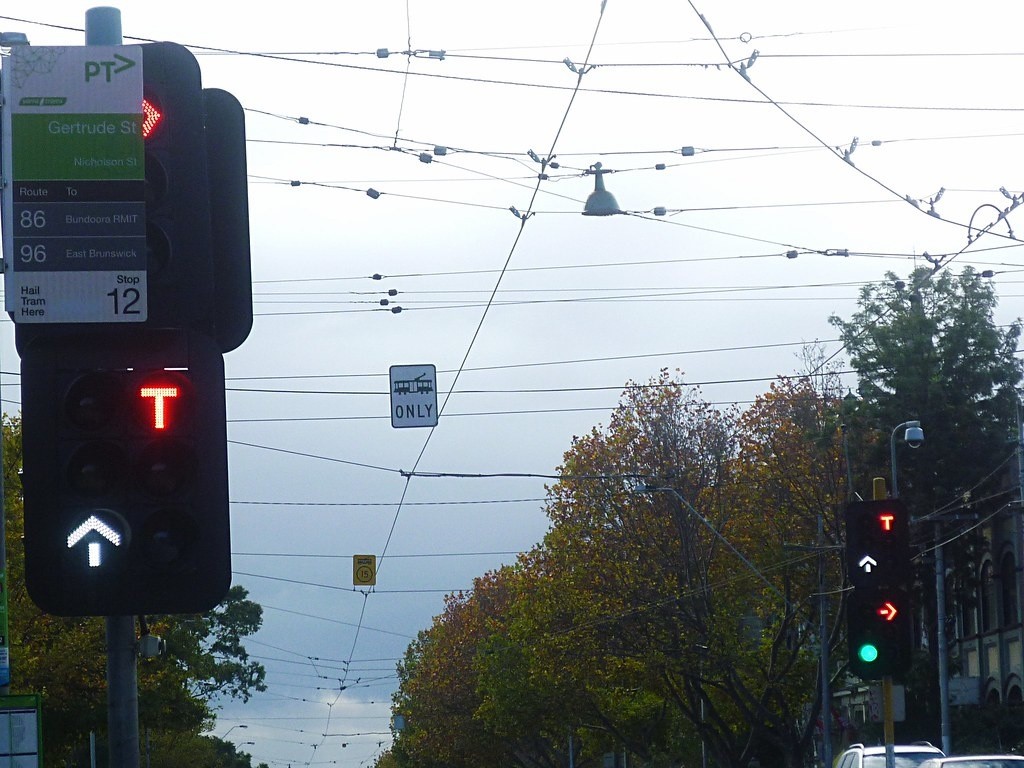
[844,499,912,673]
[0,37,233,615]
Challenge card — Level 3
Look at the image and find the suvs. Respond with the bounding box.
[837,741,945,768]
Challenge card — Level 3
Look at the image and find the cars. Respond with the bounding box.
[917,755,1024,768]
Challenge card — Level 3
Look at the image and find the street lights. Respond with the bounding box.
[873,418,925,765]
[635,485,832,767]
